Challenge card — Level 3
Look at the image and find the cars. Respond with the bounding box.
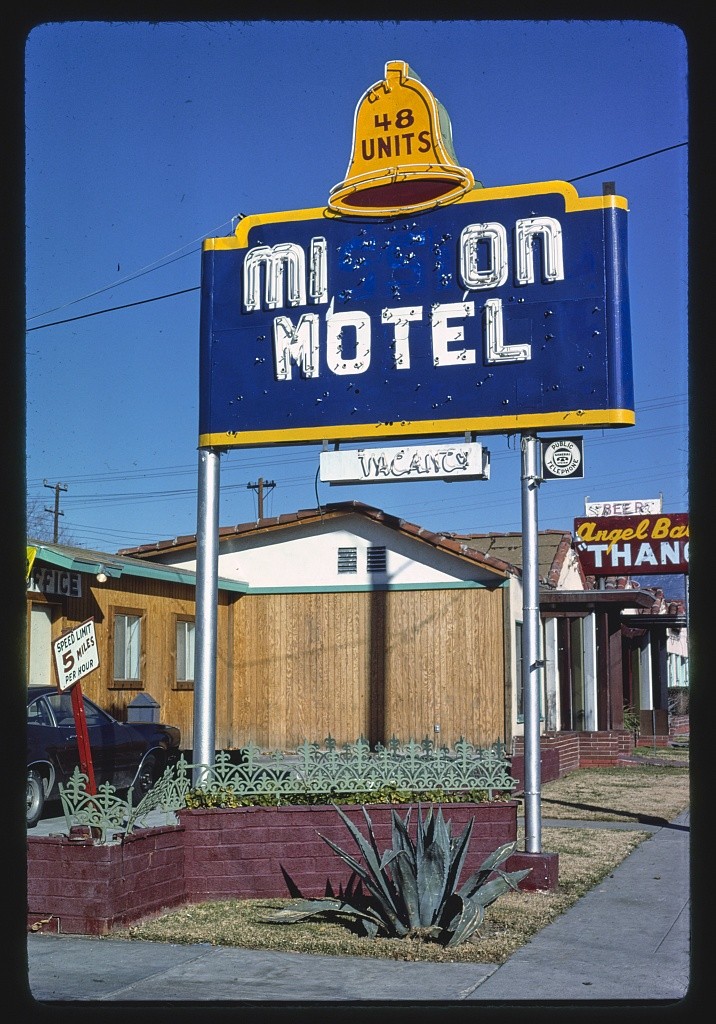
[25,683,181,828]
